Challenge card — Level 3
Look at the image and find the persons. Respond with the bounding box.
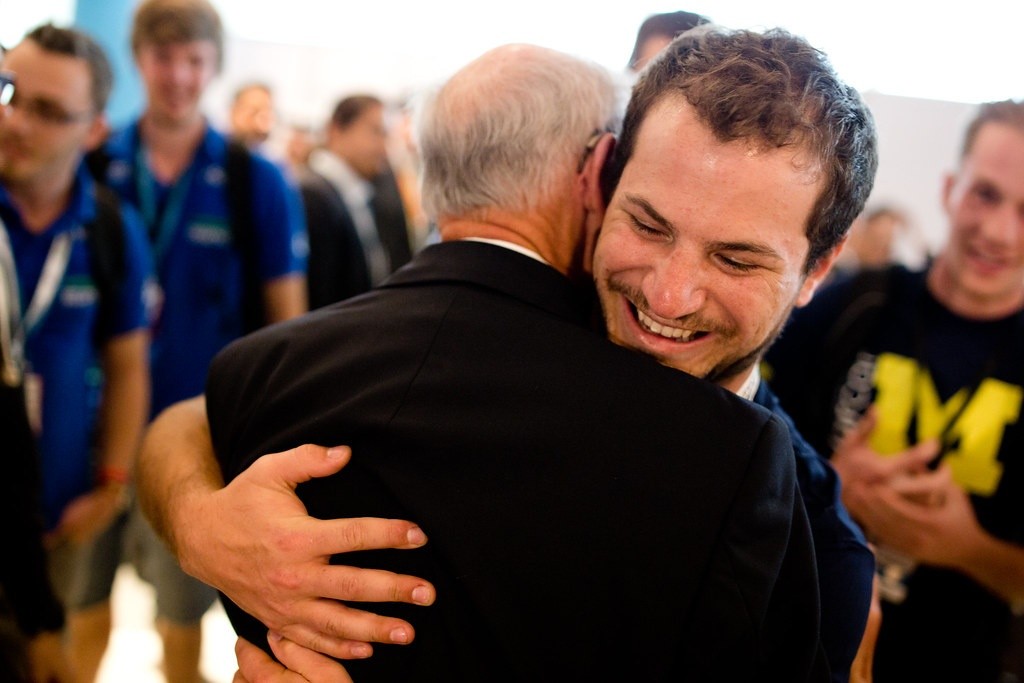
[205,41,824,680]
[0,2,925,681]
[132,24,882,681]
[757,98,1024,683]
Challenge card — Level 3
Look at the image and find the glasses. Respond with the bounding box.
[0,75,91,126]
